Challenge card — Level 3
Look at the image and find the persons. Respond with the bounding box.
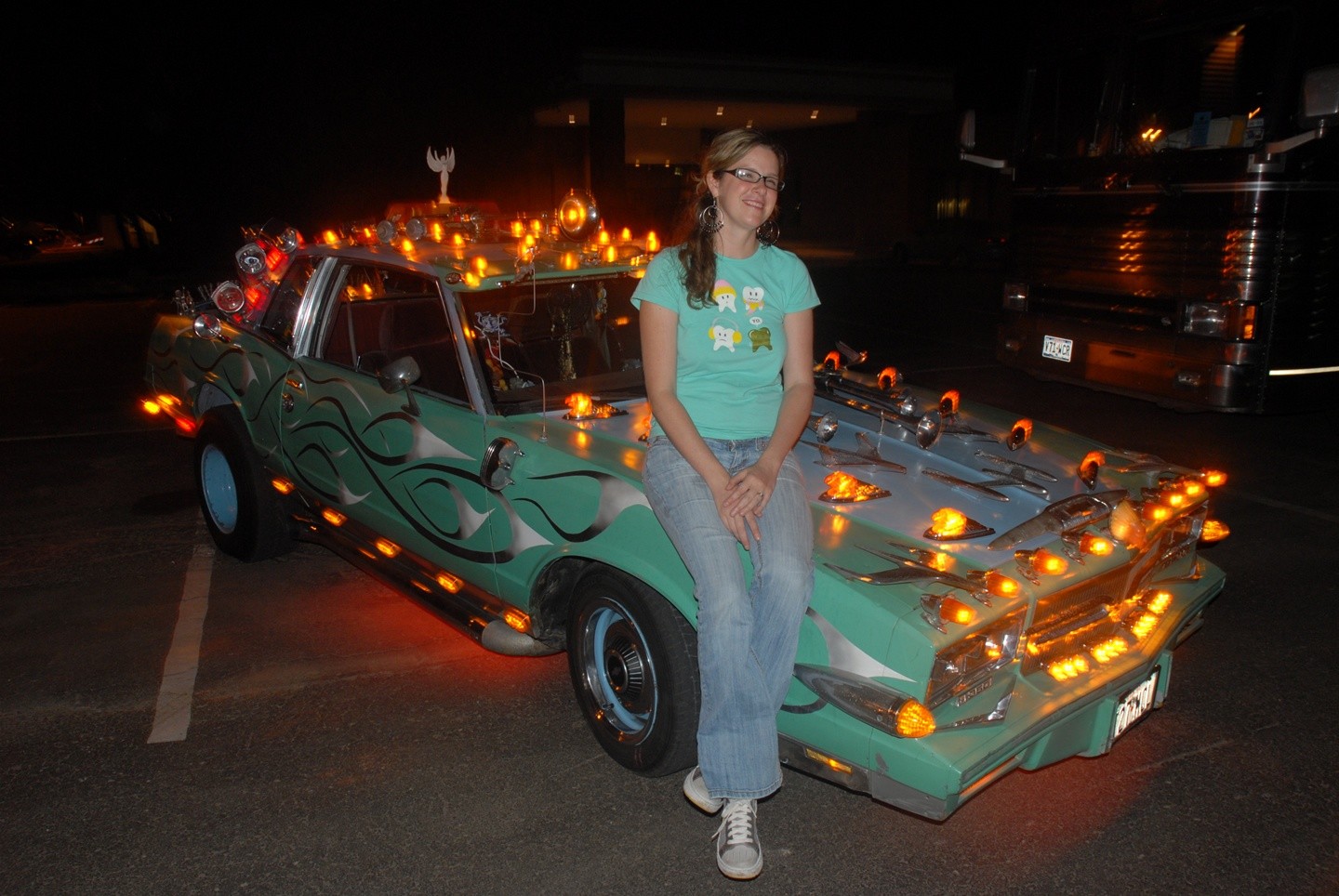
[628,129,822,882]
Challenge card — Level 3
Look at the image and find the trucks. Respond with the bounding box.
[532,26,1339,414]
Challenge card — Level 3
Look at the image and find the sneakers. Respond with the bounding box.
[683,763,723,813]
[712,798,763,880]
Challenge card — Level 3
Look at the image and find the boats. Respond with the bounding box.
[139,189,1229,822]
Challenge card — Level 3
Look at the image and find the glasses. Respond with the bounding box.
[722,168,785,191]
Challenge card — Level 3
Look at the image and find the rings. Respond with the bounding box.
[757,492,763,497]
